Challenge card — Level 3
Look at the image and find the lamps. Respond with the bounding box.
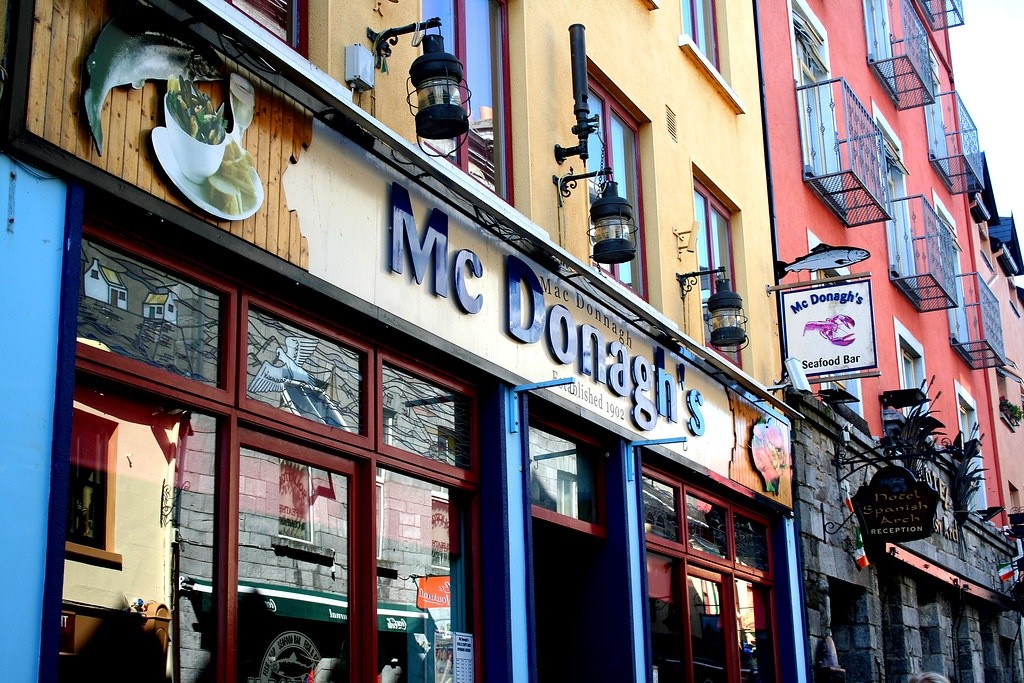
[552,166,638,265]
[952,430,984,461]
[676,266,749,353]
[998,513,1024,539]
[879,389,947,428]
[367,16,472,159]
[977,506,1004,522]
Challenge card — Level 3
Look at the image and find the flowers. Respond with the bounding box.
[999,394,1024,422]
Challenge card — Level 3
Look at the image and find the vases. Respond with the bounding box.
[1002,408,1021,426]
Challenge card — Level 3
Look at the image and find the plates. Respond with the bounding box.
[152,127,264,220]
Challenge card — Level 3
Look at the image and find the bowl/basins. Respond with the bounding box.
[165,93,226,185]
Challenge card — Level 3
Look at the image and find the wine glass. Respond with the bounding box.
[229,73,255,148]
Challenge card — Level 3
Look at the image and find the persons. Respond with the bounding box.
[436,649,453,674]
[742,633,757,669]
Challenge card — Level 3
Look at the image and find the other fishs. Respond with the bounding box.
[81,5,227,158]
[773,243,872,280]
